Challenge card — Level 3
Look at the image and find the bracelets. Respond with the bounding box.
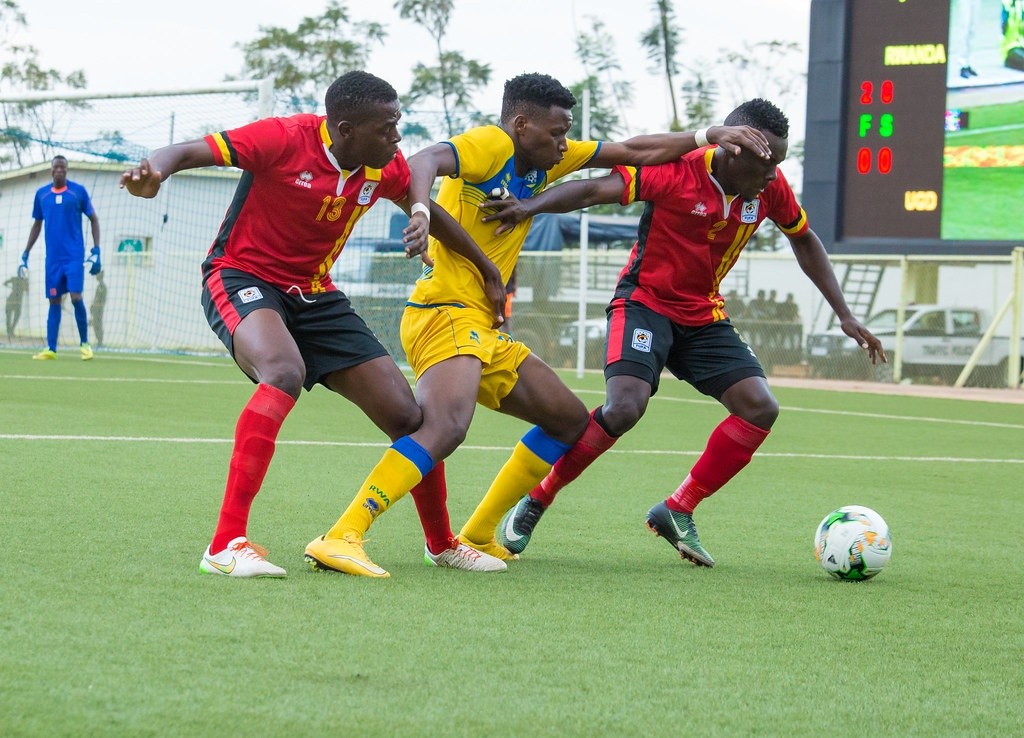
[410,202,431,224]
[694,125,715,148]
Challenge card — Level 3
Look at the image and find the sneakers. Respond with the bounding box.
[305,535,389,578]
[645,500,714,567]
[80,341,93,360]
[425,539,507,573]
[200,536,287,579]
[495,493,548,555]
[33,346,57,360]
[457,535,518,561]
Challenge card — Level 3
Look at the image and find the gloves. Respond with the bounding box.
[83,247,100,275]
[18,252,30,281]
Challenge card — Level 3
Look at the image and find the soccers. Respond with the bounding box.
[814,505,892,582]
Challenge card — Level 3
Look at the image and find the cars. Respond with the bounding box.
[556,314,607,368]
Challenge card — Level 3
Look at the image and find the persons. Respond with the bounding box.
[117,70,507,579]
[303,72,772,582]
[89,270,107,349]
[478,97,887,569]
[17,155,102,362]
[3,265,29,339]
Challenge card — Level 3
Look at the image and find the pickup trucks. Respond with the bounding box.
[804,303,1024,388]
